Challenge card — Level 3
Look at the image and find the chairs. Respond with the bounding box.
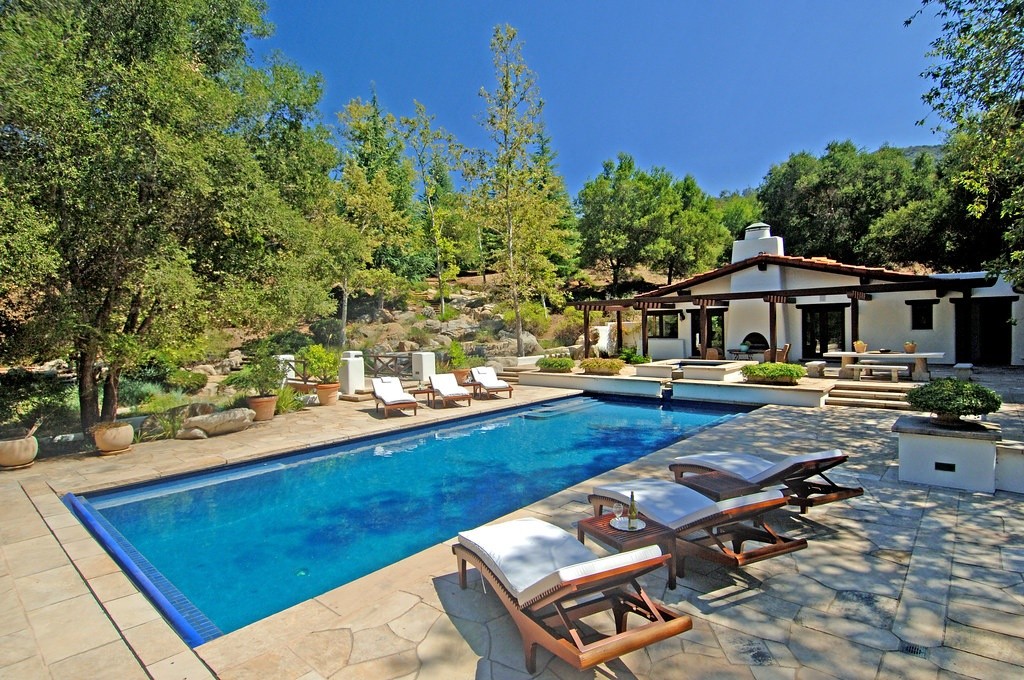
[470,366,513,399]
[429,373,472,408]
[668,448,863,528]
[698,344,724,360]
[764,343,791,363]
[587,477,808,578]
[451,515,693,676]
[371,376,418,418]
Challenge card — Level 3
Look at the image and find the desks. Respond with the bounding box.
[408,389,436,409]
[577,509,678,590]
[823,351,947,381]
[676,469,762,534]
[727,348,763,360]
[458,382,482,400]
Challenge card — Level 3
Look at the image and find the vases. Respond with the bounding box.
[855,344,868,353]
[0,436,38,471]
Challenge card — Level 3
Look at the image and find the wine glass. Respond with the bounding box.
[613,503,623,525]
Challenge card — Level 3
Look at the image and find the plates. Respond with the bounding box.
[610,517,646,531]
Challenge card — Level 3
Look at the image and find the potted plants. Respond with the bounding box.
[85,421,134,455]
[741,361,809,384]
[535,357,576,373]
[903,340,918,353]
[740,341,751,351]
[293,344,348,406]
[222,338,292,422]
[578,357,626,375]
[905,375,1002,427]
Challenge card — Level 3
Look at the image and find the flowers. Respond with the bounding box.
[854,341,864,346]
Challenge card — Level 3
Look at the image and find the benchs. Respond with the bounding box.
[953,363,974,382]
[859,360,915,377]
[845,364,908,383]
[804,361,827,377]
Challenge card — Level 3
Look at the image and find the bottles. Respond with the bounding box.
[418,380,421,390]
[628,491,637,528]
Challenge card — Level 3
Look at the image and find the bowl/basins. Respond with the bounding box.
[879,348,891,353]
